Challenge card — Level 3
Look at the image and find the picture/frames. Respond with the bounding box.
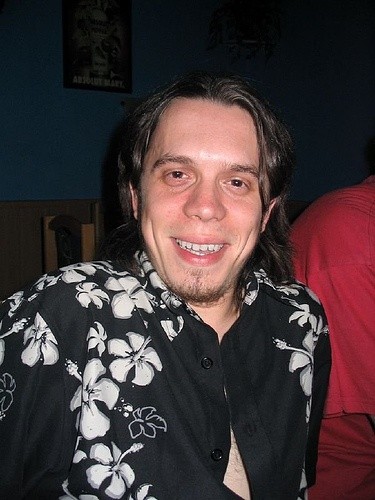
[62,0,132,95]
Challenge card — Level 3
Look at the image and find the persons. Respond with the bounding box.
[277,165,374,500]
[0,69,334,500]
